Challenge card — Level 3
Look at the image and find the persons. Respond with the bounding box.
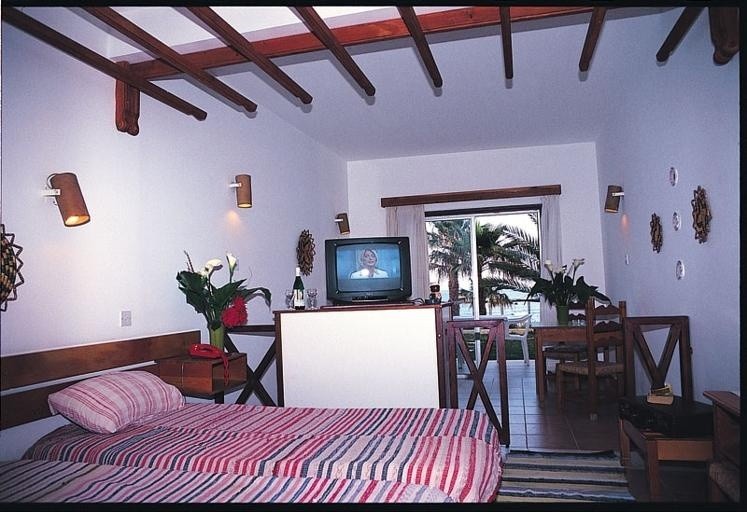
[345,247,392,281]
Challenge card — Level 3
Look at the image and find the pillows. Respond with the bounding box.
[42,367,189,438]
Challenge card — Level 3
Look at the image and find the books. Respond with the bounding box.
[646,383,674,406]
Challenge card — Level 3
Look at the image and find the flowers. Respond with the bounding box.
[174,248,273,330]
[524,252,611,312]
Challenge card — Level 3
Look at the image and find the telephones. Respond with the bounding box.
[189,344,224,358]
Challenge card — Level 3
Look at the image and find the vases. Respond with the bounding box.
[207,322,227,356]
[557,305,571,327]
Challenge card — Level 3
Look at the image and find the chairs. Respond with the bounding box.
[543,301,599,396]
[551,295,631,419]
[502,312,532,370]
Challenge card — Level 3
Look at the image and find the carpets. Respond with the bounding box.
[489,445,638,504]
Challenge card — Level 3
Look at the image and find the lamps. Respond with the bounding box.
[602,182,625,215]
[330,209,352,238]
[228,171,256,211]
[40,168,94,228]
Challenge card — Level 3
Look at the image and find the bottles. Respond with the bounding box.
[292,265,305,310]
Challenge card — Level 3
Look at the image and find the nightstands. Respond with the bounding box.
[153,350,247,407]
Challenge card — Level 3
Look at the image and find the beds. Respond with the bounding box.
[2,328,502,507]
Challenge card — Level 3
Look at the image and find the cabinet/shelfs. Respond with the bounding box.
[270,300,457,410]
[699,384,742,505]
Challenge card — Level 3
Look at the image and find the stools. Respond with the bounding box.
[615,391,714,501]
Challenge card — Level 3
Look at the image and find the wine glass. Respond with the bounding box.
[306,289,317,311]
[284,289,292,311]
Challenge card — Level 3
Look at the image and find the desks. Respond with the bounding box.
[530,322,626,401]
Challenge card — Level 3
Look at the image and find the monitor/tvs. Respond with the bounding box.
[324,237,411,304]
